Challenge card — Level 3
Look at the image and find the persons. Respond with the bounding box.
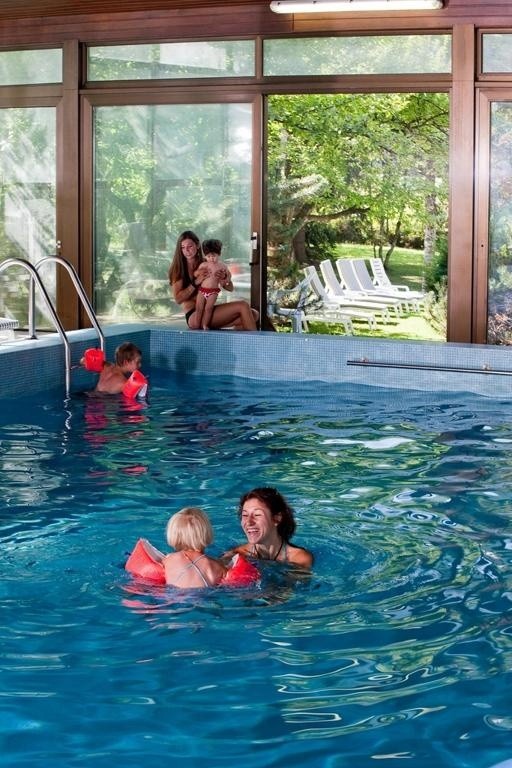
[223,485,313,571]
[80,340,143,396]
[193,239,227,330]
[159,505,227,588]
[168,229,259,331]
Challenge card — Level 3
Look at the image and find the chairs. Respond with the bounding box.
[267,257,423,336]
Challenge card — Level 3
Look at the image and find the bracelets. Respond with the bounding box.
[191,281,197,289]
